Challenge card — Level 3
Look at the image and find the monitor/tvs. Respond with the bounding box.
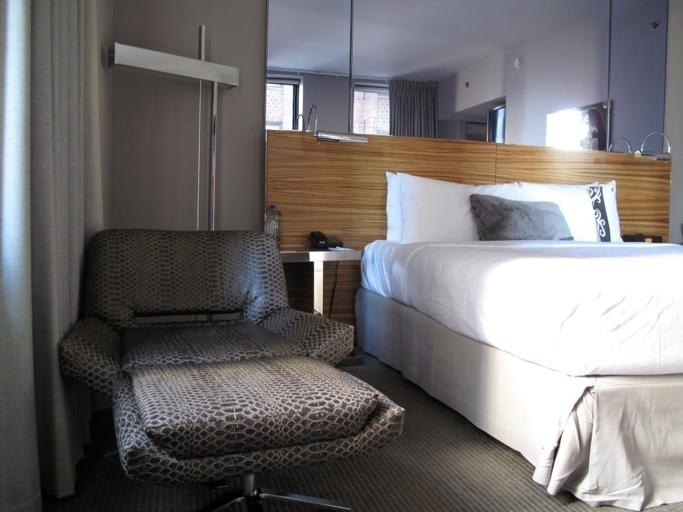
[544,100,615,153]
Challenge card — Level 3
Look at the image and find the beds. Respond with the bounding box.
[268,130,683,512]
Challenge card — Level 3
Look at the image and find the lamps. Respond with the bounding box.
[307,104,368,144]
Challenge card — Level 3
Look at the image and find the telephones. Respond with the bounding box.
[310,231,342,250]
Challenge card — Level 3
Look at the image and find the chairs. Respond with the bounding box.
[58,231,407,512]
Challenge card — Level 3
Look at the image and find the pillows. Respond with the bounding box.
[387,171,623,242]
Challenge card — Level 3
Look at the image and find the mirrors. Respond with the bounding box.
[267,0,669,154]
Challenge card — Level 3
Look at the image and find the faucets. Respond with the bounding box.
[296,114,304,131]
[609,137,632,154]
[307,104,318,138]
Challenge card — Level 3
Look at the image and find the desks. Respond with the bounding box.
[280,251,365,314]
